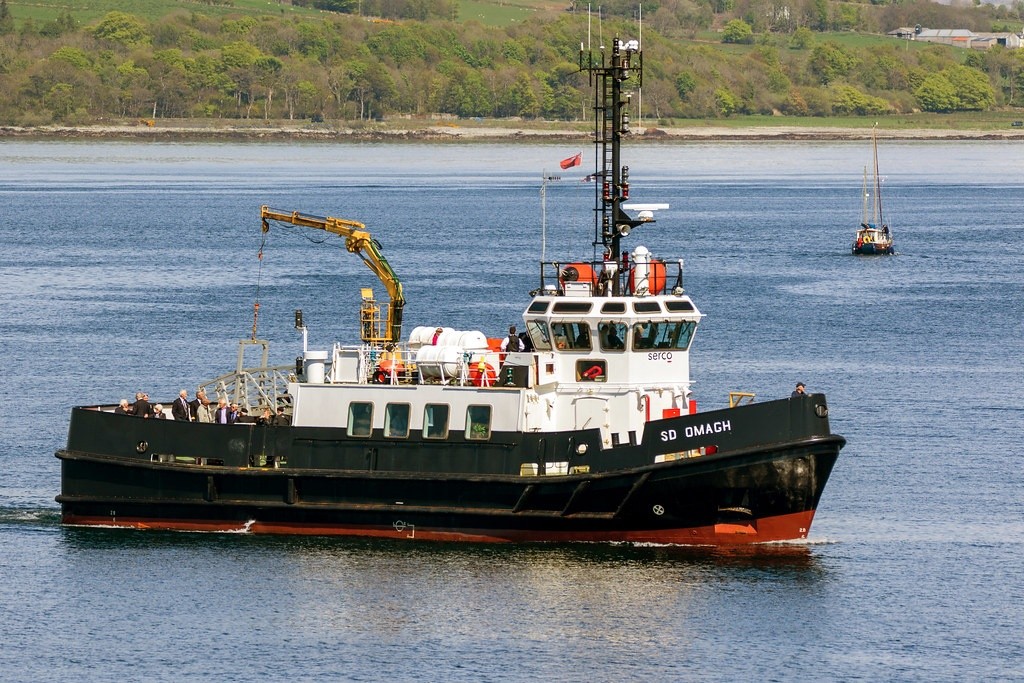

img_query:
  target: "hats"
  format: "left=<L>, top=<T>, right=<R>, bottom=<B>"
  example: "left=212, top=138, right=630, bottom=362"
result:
left=796, top=382, right=806, bottom=387
left=155, top=404, right=162, bottom=412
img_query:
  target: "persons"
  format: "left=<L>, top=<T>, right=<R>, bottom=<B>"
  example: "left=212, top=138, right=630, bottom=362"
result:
left=190, top=388, right=292, bottom=427
left=500, top=326, right=525, bottom=353
left=392, top=407, right=407, bottom=436
left=172, top=390, right=190, bottom=422
left=792, top=382, right=806, bottom=396
left=115, top=393, right=166, bottom=420
left=576, top=325, right=645, bottom=349
left=882, top=225, right=889, bottom=241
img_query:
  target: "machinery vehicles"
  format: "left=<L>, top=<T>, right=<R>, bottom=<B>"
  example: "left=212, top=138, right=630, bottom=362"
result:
left=251, top=202, right=419, bottom=383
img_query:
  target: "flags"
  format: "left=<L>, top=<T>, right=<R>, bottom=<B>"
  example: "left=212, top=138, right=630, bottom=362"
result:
left=560, top=152, right=582, bottom=169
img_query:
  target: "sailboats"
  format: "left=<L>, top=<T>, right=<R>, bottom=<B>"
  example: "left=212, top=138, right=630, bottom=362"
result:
left=847, top=122, right=895, bottom=256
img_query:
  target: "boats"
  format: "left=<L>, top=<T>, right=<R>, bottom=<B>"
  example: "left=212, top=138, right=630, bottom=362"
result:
left=52, top=0, right=850, bottom=558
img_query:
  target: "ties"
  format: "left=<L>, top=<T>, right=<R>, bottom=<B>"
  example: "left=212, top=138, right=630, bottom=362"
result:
left=232, top=413, right=234, bottom=420
left=183, top=401, right=187, bottom=416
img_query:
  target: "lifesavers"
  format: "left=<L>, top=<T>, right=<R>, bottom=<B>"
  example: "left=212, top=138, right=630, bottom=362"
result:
left=858, top=237, right=862, bottom=248
left=583, top=365, right=602, bottom=379
left=863, top=235, right=870, bottom=244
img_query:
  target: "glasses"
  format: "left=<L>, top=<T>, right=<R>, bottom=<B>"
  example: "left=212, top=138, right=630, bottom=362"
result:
left=143, top=395, right=149, bottom=397
